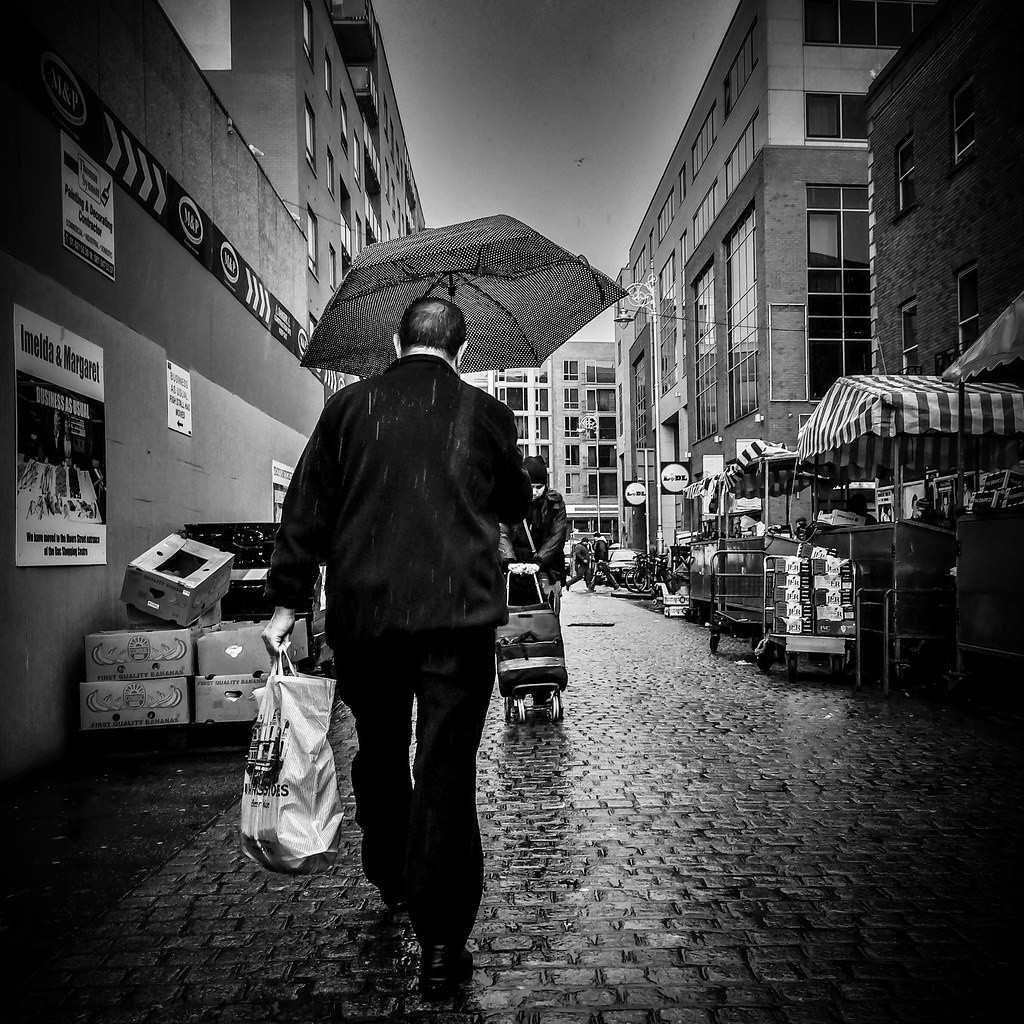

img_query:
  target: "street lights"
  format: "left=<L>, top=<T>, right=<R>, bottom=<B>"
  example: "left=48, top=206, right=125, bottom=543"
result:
left=614, top=258, right=660, bottom=599
left=576, top=404, right=601, bottom=532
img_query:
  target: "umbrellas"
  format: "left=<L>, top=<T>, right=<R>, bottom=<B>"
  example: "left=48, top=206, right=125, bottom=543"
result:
left=296, top=213, right=631, bottom=374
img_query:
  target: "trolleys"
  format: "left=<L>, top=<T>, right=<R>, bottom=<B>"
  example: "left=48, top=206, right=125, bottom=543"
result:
left=708, top=550, right=775, bottom=653
left=756, top=553, right=856, bottom=675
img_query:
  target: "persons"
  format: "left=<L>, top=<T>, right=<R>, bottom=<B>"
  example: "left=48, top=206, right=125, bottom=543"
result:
left=564, top=536, right=597, bottom=592
left=495, top=453, right=568, bottom=717
left=793, top=516, right=807, bottom=531
left=878, top=478, right=972, bottom=524
left=583, top=531, right=622, bottom=594
left=647, top=539, right=657, bottom=555
left=262, top=291, right=533, bottom=996
left=844, top=492, right=877, bottom=525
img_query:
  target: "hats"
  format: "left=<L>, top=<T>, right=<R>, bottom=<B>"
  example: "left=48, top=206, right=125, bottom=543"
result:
left=582, top=537, right=590, bottom=542
left=524, top=456, right=548, bottom=485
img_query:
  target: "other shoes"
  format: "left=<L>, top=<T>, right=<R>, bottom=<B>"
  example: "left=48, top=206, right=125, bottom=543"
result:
left=614, top=586, right=621, bottom=591
left=584, top=588, right=594, bottom=593
left=566, top=582, right=570, bottom=591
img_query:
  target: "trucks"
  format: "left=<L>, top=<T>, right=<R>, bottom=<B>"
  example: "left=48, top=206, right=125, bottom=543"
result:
left=568, top=533, right=613, bottom=560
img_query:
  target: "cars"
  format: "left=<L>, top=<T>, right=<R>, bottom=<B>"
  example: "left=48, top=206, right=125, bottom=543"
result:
left=601, top=548, right=639, bottom=585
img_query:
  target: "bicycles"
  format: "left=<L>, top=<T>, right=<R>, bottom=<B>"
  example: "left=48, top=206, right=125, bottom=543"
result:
left=625, top=552, right=674, bottom=594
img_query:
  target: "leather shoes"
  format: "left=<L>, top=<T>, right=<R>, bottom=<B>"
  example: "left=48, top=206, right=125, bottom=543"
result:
left=421, top=945, right=473, bottom=1000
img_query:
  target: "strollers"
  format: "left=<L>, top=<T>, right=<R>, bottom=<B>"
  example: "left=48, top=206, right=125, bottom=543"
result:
left=500, top=569, right=561, bottom=725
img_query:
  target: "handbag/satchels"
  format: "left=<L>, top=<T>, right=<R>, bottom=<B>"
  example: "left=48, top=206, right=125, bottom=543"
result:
left=595, top=562, right=610, bottom=585
left=240, top=643, right=344, bottom=876
